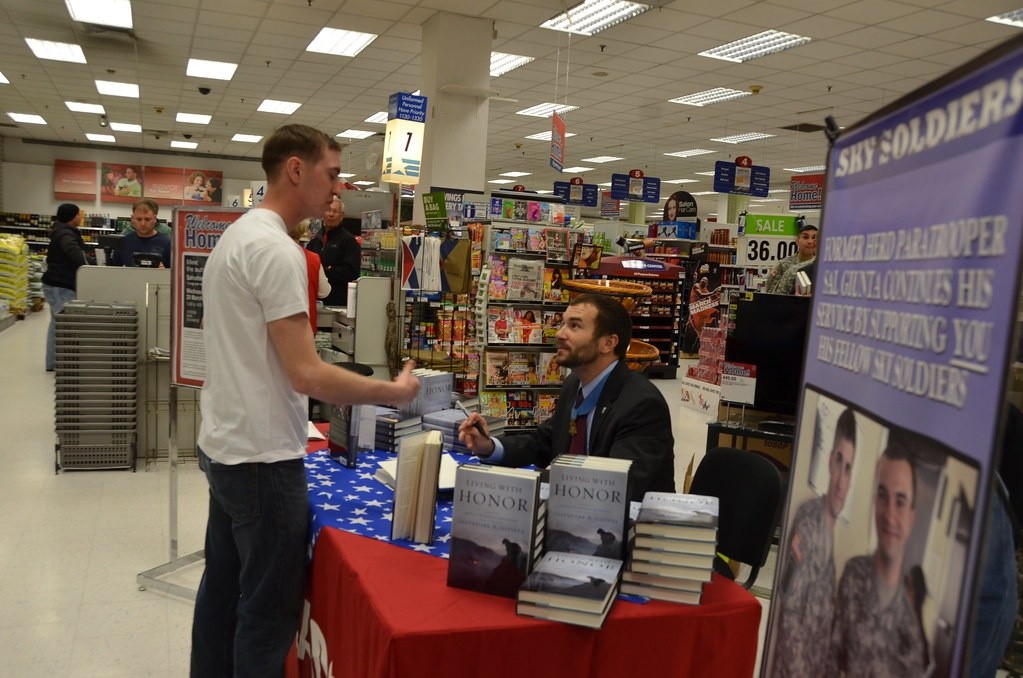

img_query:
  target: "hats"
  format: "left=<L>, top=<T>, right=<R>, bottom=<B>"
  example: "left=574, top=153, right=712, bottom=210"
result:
left=52, top=203, right=79, bottom=223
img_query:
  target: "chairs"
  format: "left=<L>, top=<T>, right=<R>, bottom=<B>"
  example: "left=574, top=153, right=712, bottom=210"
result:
left=688, top=445, right=786, bottom=592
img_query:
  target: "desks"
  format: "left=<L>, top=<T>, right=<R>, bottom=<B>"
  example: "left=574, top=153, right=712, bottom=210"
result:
left=283, top=422, right=762, bottom=678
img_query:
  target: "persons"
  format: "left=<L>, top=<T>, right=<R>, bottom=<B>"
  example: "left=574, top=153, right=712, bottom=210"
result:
left=554, top=312, right=565, bottom=327
left=496, top=312, right=512, bottom=341
left=41, top=203, right=92, bottom=373
left=767, top=224, right=819, bottom=295
left=546, top=356, right=562, bottom=383
left=524, top=362, right=536, bottom=382
left=664, top=197, right=678, bottom=223
left=305, top=197, right=361, bottom=306
left=521, top=311, right=536, bottom=343
left=187, top=123, right=421, bottom=678
left=458, top=293, right=676, bottom=501
left=288, top=217, right=332, bottom=421
left=545, top=315, right=553, bottom=327
left=103, top=166, right=141, bottom=197
left=830, top=441, right=931, bottom=678
left=688, top=277, right=720, bottom=351
left=769, top=408, right=857, bottom=678
left=106, top=200, right=172, bottom=270
left=189, top=171, right=221, bottom=202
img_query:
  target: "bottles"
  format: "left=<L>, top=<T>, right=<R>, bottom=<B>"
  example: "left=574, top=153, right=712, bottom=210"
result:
left=0, top=211, right=110, bottom=262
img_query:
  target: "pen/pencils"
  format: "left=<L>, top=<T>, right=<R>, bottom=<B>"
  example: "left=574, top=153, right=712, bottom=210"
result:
left=456, top=400, right=489, bottom=440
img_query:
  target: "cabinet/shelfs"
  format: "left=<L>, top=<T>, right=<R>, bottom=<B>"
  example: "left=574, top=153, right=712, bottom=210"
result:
left=588, top=256, right=686, bottom=380
left=718, top=264, right=760, bottom=324
left=644, top=238, right=700, bottom=258
left=482, top=223, right=586, bottom=433
left=0, top=224, right=115, bottom=247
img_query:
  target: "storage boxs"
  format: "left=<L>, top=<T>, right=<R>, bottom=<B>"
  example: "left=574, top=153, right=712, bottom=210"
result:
left=477, top=229, right=728, bottom=423
left=51, top=300, right=139, bottom=471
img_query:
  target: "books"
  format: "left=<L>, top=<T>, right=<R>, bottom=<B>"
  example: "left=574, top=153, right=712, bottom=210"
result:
left=447, top=462, right=551, bottom=597
left=390, top=427, right=444, bottom=545
left=795, top=270, right=812, bottom=294
left=479, top=200, right=584, bottom=426
left=328, top=367, right=475, bottom=500
left=706, top=249, right=732, bottom=264
left=704, top=227, right=730, bottom=245
left=620, top=490, right=720, bottom=606
left=718, top=268, right=741, bottom=285
left=545, top=454, right=635, bottom=558
left=516, top=549, right=623, bottom=629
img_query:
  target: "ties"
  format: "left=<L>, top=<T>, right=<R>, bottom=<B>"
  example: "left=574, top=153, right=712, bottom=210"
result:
left=569, top=388, right=589, bottom=455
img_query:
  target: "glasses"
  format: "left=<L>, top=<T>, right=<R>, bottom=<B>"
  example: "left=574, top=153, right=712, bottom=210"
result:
left=327, top=209, right=342, bottom=215
